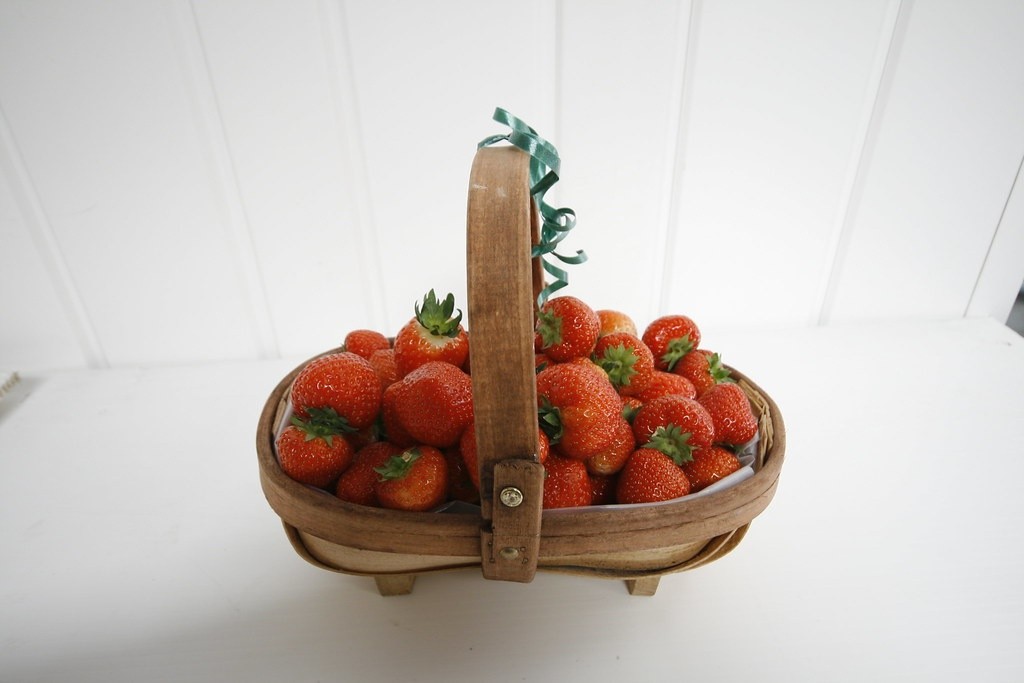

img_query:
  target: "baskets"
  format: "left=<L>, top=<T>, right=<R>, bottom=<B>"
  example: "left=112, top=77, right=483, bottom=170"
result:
left=254, top=144, right=786, bottom=594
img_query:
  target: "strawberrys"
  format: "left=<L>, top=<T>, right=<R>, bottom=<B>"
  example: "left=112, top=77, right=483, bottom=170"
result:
left=272, top=289, right=756, bottom=512
